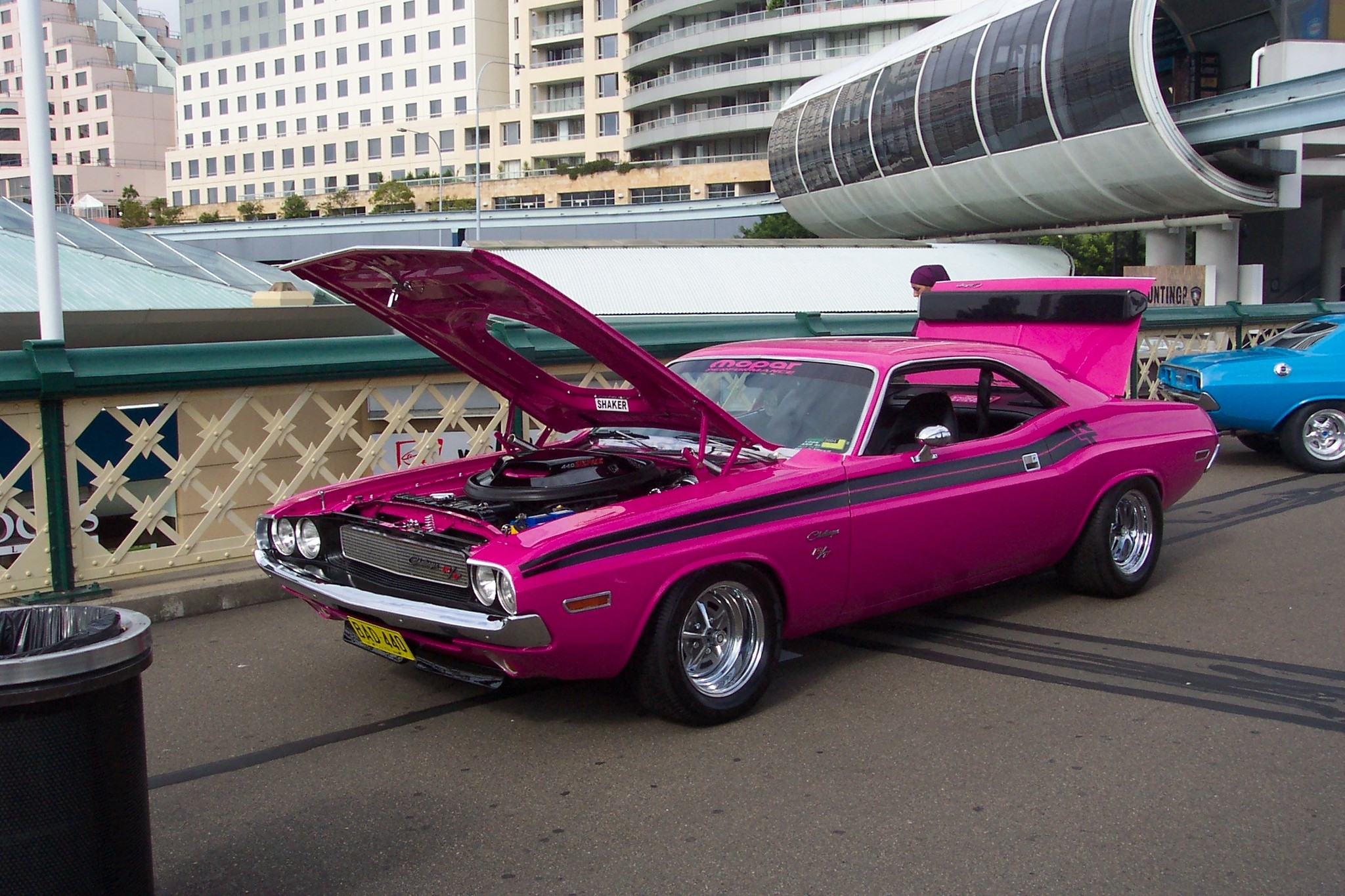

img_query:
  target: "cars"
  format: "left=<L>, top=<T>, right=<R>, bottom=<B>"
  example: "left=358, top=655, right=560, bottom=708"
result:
left=250, top=243, right=1223, bottom=731
left=1156, top=312, right=1345, bottom=475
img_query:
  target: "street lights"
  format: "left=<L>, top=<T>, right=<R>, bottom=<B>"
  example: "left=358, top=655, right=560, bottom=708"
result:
left=19, top=184, right=114, bottom=215
left=475, top=60, right=526, bottom=245
left=397, top=127, right=442, bottom=213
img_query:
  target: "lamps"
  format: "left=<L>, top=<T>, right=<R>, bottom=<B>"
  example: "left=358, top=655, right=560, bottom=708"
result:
left=483, top=202, right=488, bottom=206
left=694, top=189, right=700, bottom=194
left=548, top=198, right=553, bottom=202
left=618, top=193, right=623, bottom=198
left=416, top=206, right=422, bottom=210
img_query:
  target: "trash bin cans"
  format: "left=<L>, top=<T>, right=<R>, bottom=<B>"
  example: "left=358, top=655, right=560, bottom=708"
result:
left=0, top=604, right=155, bottom=896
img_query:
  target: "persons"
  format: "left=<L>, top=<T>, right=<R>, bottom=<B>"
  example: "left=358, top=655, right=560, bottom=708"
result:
left=910, top=265, right=950, bottom=299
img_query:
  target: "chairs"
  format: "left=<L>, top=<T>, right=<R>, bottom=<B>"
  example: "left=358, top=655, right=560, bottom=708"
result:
left=877, top=392, right=959, bottom=455
